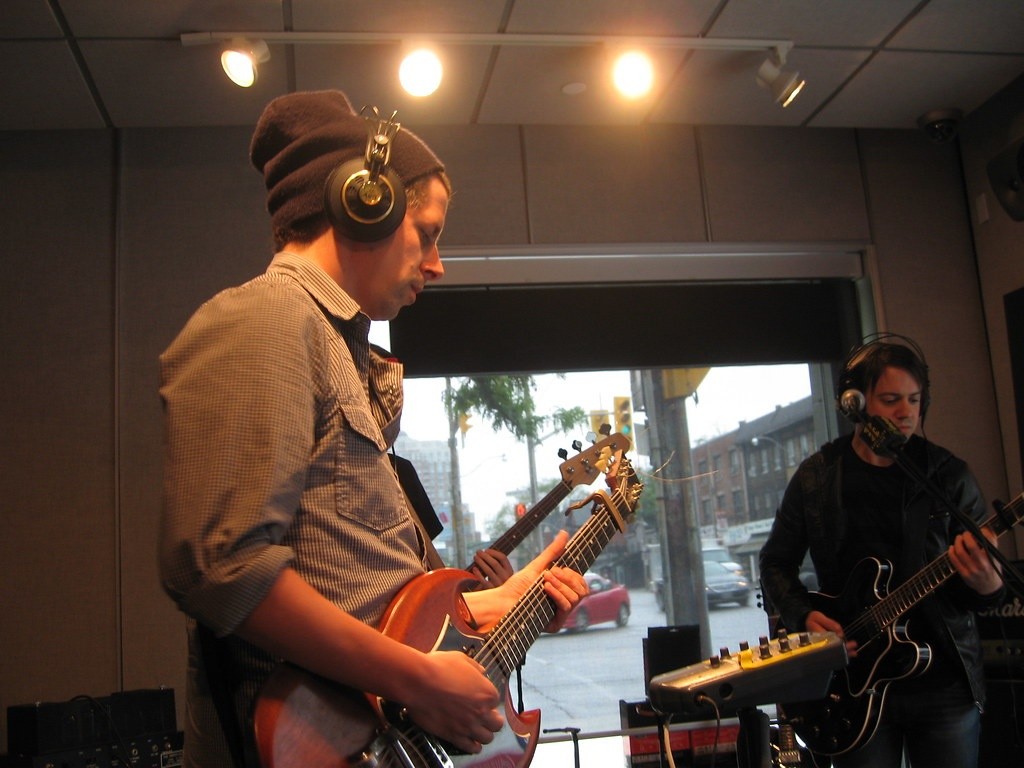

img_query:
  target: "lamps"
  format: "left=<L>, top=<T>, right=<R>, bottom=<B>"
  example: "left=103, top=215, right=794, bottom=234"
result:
left=219, top=32, right=274, bottom=88
left=751, top=49, right=803, bottom=106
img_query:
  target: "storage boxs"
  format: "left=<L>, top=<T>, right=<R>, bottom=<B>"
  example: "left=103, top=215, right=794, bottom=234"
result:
left=618, top=699, right=740, bottom=768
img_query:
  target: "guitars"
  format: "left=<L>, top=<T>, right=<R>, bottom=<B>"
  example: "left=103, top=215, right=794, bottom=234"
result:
left=461, top=422, right=630, bottom=577
left=253, top=445, right=644, bottom=768
left=768, top=493, right=1024, bottom=756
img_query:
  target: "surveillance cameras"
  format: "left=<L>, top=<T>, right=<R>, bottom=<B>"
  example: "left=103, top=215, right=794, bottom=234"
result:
left=916, top=107, right=964, bottom=143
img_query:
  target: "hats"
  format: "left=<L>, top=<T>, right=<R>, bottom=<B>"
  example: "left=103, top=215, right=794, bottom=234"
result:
left=248, top=90, right=446, bottom=235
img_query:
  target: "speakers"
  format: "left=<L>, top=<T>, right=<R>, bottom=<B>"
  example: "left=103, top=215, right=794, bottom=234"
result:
left=978, top=679, right=1024, bottom=768
left=642, top=625, right=702, bottom=695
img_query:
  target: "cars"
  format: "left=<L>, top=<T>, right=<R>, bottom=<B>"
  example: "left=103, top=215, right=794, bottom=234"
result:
left=563, top=572, right=631, bottom=633
left=654, top=559, right=750, bottom=613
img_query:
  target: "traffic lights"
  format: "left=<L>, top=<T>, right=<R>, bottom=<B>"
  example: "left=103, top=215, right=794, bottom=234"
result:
left=515, top=503, right=526, bottom=520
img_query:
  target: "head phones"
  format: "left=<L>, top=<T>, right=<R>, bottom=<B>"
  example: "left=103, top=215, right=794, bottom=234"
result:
left=836, top=331, right=930, bottom=423
left=325, top=103, right=406, bottom=242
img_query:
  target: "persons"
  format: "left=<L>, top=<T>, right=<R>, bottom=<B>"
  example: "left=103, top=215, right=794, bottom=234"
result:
left=154, top=91, right=589, bottom=768
left=760, top=342, right=1004, bottom=768
